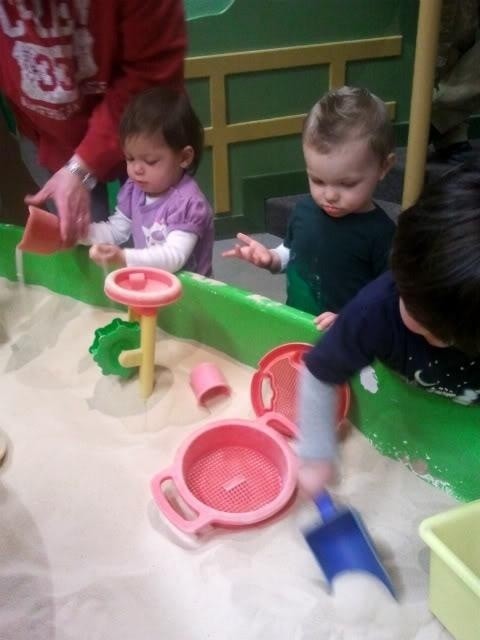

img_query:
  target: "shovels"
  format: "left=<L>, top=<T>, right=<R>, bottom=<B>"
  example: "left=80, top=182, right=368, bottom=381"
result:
left=18, top=205, right=63, bottom=254
left=302, top=490, right=398, bottom=602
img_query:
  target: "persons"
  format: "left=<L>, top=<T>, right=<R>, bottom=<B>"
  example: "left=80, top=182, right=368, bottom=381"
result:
left=76, top=88, right=216, bottom=276
left=294, top=157, right=480, bottom=499
left=220, top=85, right=397, bottom=333
left=0, top=0, right=188, bottom=250
